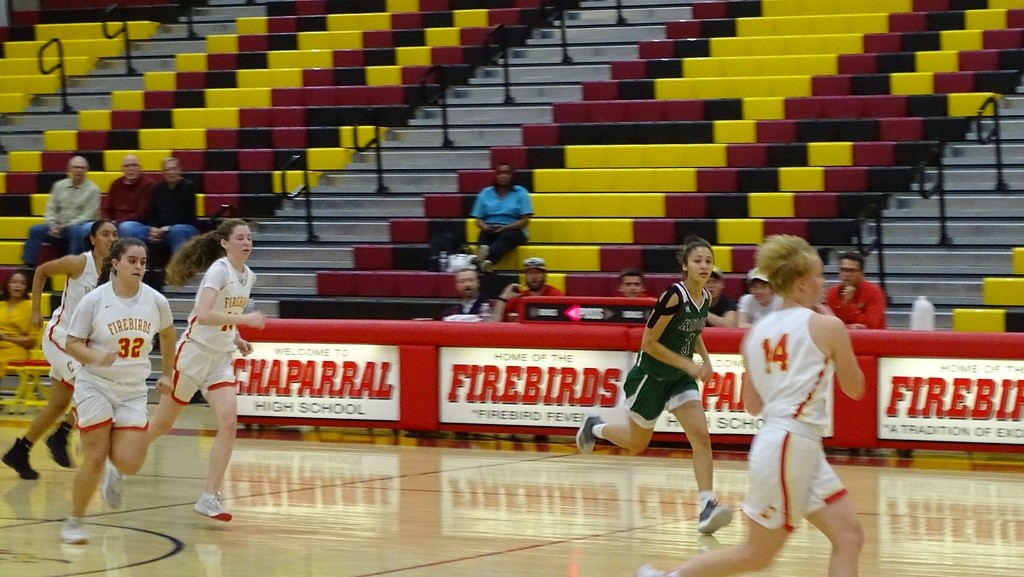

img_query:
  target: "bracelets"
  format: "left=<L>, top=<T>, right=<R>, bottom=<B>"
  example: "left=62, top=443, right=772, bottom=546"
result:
left=496, top=297, right=508, bottom=303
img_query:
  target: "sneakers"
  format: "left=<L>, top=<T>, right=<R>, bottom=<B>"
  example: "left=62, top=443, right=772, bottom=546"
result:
left=45, top=428, right=70, bottom=469
left=697, top=499, right=733, bottom=532
left=194, top=492, right=232, bottom=522
left=3, top=437, right=39, bottom=481
left=102, top=458, right=124, bottom=509
left=60, top=518, right=88, bottom=545
left=576, top=412, right=604, bottom=454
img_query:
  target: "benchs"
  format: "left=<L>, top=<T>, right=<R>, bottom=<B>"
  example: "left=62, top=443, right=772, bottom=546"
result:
left=0, top=0, right=1024, bottom=321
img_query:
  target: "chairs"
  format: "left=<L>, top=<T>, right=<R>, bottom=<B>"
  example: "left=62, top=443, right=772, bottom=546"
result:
left=1, top=322, right=54, bottom=414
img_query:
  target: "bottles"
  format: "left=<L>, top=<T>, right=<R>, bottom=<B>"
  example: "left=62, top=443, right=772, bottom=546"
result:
left=910, top=296, right=936, bottom=330
left=439, top=252, right=447, bottom=272
left=479, top=302, right=492, bottom=322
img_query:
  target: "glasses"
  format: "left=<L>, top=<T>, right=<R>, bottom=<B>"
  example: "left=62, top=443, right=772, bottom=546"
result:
left=839, top=266, right=862, bottom=274
left=525, top=260, right=543, bottom=266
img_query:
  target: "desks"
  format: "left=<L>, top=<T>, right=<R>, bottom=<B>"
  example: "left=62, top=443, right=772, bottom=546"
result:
left=236, top=321, right=1024, bottom=459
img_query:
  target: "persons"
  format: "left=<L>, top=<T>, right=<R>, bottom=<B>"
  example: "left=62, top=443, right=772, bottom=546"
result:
left=826, top=252, right=887, bottom=329
left=0, top=270, right=39, bottom=381
left=147, top=218, right=267, bottom=521
left=440, top=269, right=490, bottom=321
left=471, top=163, right=534, bottom=273
left=636, top=236, right=865, bottom=577
left=2, top=220, right=120, bottom=481
left=490, top=257, right=562, bottom=323
left=21, top=156, right=101, bottom=293
left=120, top=159, right=201, bottom=270
left=78, top=155, right=159, bottom=255
left=60, top=239, right=177, bottom=544
left=737, top=267, right=784, bottom=328
left=704, top=268, right=737, bottom=328
left=575, top=237, right=733, bottom=534
left=617, top=269, right=648, bottom=298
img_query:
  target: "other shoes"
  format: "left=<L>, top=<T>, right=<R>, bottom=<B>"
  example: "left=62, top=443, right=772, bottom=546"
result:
left=470, top=257, right=493, bottom=272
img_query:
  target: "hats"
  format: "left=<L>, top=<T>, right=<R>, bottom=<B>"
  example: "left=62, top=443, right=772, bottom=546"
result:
left=709, top=264, right=721, bottom=279
left=524, top=256, right=548, bottom=272
left=746, top=267, right=770, bottom=283
